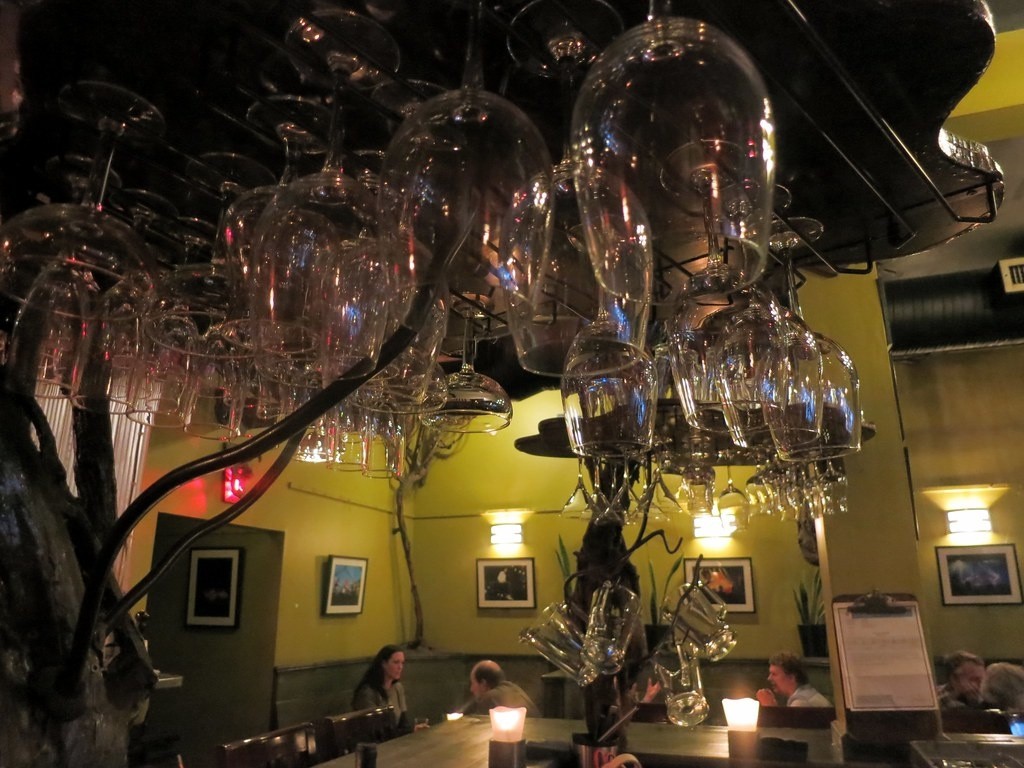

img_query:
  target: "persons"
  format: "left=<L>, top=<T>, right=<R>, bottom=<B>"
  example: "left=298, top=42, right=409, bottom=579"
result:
left=622, top=657, right=662, bottom=703
left=935, top=650, right=1024, bottom=734
left=486, top=567, right=517, bottom=600
left=756, top=650, right=834, bottom=707
left=351, top=645, right=430, bottom=731
left=455, top=660, right=543, bottom=719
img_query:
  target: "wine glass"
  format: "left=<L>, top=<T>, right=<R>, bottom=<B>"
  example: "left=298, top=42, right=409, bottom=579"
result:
left=0, top=0, right=861, bottom=525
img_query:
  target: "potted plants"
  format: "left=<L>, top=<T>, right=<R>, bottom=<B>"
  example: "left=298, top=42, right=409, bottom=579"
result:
left=792, top=567, right=827, bottom=659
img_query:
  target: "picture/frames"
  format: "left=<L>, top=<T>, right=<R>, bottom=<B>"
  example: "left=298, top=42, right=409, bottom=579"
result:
left=322, top=555, right=369, bottom=615
left=683, top=557, right=756, bottom=614
left=935, top=544, right=1024, bottom=607
left=184, top=547, right=244, bottom=629
left=476, top=557, right=537, bottom=609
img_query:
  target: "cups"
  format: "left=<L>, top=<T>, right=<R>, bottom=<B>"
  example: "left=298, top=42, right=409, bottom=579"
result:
left=517, top=584, right=641, bottom=687
left=651, top=583, right=739, bottom=727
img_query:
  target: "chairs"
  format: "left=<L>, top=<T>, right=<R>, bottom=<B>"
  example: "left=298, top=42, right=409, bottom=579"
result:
left=148, top=706, right=398, bottom=768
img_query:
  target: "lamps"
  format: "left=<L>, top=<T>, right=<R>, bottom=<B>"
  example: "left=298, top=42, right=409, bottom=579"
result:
left=489, top=524, right=523, bottom=544
left=489, top=705, right=529, bottom=768
left=946, top=506, right=994, bottom=534
left=693, top=514, right=732, bottom=538
left=721, top=699, right=759, bottom=757
